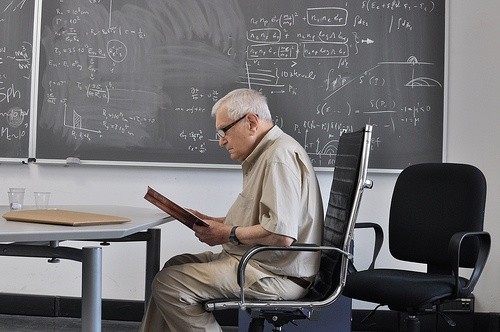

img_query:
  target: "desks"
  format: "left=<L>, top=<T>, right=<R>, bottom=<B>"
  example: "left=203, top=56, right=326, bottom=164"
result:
left=0, top=205, right=177, bottom=332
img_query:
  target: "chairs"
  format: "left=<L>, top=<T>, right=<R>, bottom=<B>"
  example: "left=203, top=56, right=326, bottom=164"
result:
left=201, top=124, right=374, bottom=332
left=341, top=162, right=491, bottom=332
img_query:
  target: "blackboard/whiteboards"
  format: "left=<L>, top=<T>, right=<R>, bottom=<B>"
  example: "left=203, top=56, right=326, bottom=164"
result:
left=0, top=0, right=39, bottom=162
left=30, top=0, right=448, bottom=173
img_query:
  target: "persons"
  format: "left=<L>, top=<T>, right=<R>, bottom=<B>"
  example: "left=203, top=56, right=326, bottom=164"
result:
left=138, top=88, right=324, bottom=332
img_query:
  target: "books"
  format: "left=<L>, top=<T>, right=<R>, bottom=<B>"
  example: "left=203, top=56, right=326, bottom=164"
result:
left=144, top=185, right=210, bottom=232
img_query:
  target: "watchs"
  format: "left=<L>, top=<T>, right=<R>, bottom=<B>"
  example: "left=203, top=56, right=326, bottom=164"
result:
left=229, top=226, right=241, bottom=246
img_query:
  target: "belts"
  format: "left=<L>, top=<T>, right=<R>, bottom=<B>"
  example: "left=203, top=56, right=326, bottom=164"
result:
left=282, top=275, right=314, bottom=289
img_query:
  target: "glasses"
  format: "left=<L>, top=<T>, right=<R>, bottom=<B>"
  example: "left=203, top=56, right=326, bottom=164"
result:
left=215, top=113, right=258, bottom=140
